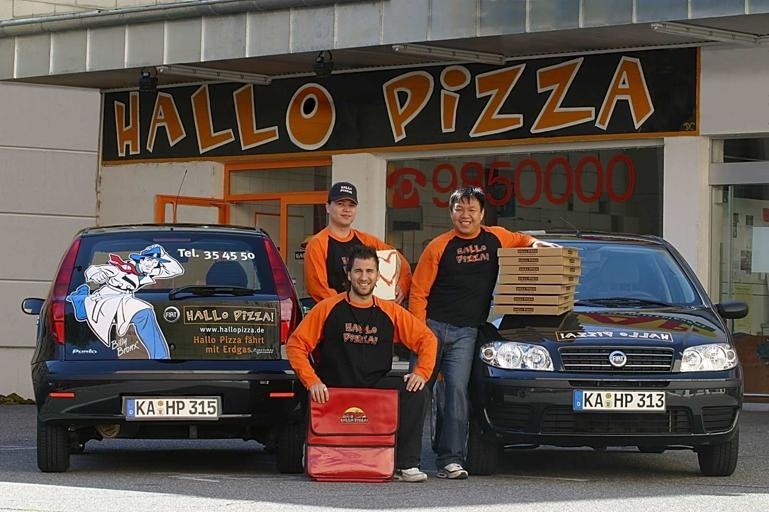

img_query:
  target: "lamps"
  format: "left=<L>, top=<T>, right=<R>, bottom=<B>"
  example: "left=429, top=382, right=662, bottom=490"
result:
left=651, top=21, right=758, bottom=48
left=313, top=50, right=335, bottom=78
left=139, top=66, right=158, bottom=92
left=156, top=65, right=272, bottom=86
left=392, top=44, right=505, bottom=66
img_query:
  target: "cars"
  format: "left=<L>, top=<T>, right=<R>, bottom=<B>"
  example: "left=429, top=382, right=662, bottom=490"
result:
left=429, top=230, right=748, bottom=476
left=20, top=221, right=316, bottom=475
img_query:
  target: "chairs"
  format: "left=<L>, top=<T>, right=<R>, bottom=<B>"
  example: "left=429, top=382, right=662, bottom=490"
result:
left=599, top=253, right=643, bottom=297
left=206, top=261, right=248, bottom=287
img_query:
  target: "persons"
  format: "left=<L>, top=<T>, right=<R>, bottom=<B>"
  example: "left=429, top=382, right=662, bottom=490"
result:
left=287, top=245, right=441, bottom=484
left=408, top=183, right=564, bottom=479
left=303, top=182, right=413, bottom=311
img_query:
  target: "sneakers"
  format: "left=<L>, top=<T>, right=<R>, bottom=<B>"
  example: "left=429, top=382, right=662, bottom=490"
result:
left=437, top=463, right=468, bottom=479
left=395, top=466, right=426, bottom=483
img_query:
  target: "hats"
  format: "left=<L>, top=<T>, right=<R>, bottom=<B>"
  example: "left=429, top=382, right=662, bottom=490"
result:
left=329, top=182, right=358, bottom=205
left=131, top=246, right=169, bottom=265
